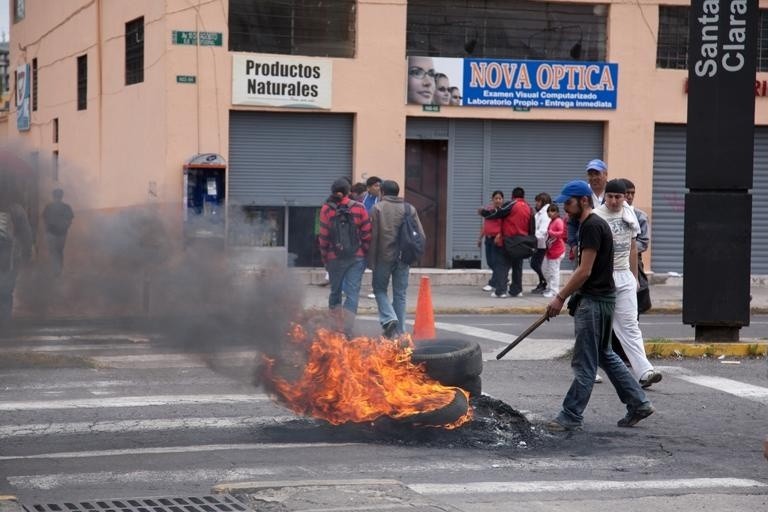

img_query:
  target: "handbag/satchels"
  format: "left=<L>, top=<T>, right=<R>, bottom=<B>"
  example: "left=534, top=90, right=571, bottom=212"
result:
left=503, top=235, right=538, bottom=260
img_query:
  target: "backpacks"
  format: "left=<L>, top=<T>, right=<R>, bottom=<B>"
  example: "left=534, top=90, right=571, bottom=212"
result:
left=395, top=202, right=427, bottom=264
left=326, top=200, right=361, bottom=259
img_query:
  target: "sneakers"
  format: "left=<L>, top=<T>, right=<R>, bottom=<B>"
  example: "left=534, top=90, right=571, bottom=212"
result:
left=638, top=370, right=663, bottom=389
left=483, top=285, right=522, bottom=298
left=616, top=403, right=655, bottom=427
left=547, top=420, right=568, bottom=431
left=383, top=320, right=396, bottom=339
left=532, top=287, right=557, bottom=297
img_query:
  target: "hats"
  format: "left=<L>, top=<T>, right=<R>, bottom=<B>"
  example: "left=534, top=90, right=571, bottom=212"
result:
left=553, top=179, right=591, bottom=204
left=585, top=158, right=607, bottom=173
left=605, top=179, right=627, bottom=194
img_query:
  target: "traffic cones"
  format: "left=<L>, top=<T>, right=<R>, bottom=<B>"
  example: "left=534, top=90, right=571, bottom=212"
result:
left=412, top=276, right=436, bottom=340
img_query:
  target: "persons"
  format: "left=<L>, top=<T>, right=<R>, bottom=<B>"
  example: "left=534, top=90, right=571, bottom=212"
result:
left=368, top=180, right=427, bottom=339
left=449, top=87, right=461, bottom=106
left=351, top=177, right=383, bottom=273
left=319, top=177, right=372, bottom=342
left=478, top=186, right=536, bottom=297
left=434, top=73, right=451, bottom=104
left=530, top=192, right=553, bottom=293
left=566, top=217, right=581, bottom=272
left=43, top=188, right=73, bottom=270
left=476, top=190, right=504, bottom=291
left=540, top=203, right=567, bottom=297
left=592, top=179, right=663, bottom=388
left=546, top=180, right=656, bottom=431
left=1, top=189, right=34, bottom=326
left=408, top=57, right=435, bottom=105
left=587, top=159, right=611, bottom=209
